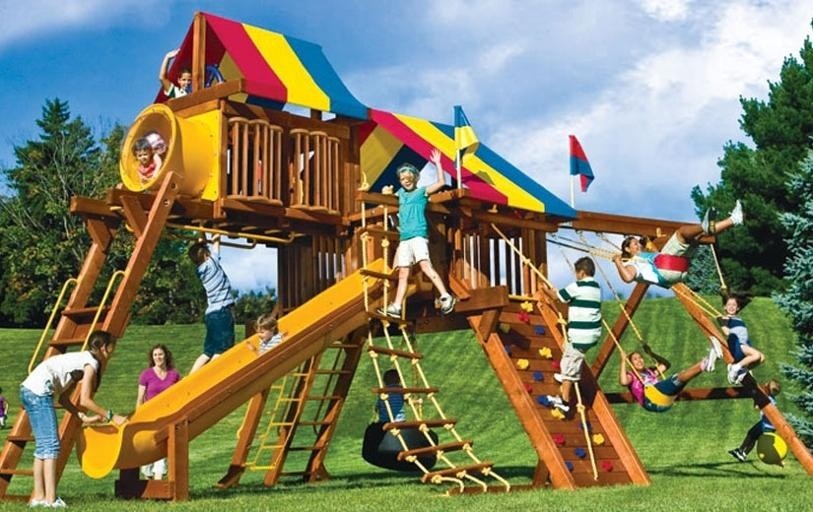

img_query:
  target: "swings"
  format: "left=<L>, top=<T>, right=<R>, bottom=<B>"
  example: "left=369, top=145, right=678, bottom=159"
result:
left=598, top=233, right=751, bottom=362
left=541, top=233, right=690, bottom=286
left=709, top=244, right=789, bottom=463
left=553, top=233, right=679, bottom=413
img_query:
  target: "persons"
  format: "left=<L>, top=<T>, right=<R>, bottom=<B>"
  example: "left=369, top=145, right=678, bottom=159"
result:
left=541, top=257, right=601, bottom=412
left=369, top=369, right=422, bottom=427
left=728, top=382, right=781, bottom=463
left=717, top=293, right=765, bottom=385
left=184, top=221, right=235, bottom=379
left=612, top=199, right=745, bottom=291
left=19, top=329, right=128, bottom=507
left=131, top=138, right=156, bottom=183
left=158, top=48, right=192, bottom=100
left=143, top=130, right=169, bottom=176
left=136, top=342, right=181, bottom=480
left=619, top=335, right=723, bottom=412
left=247, top=315, right=286, bottom=360
left=0, top=388, right=9, bottom=429
left=376, top=148, right=456, bottom=319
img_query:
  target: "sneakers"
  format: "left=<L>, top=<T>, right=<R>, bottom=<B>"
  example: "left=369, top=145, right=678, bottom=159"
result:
left=375, top=302, right=402, bottom=319
left=554, top=373, right=564, bottom=384
left=547, top=394, right=571, bottom=412
left=440, top=293, right=457, bottom=315
left=708, top=335, right=724, bottom=361
left=726, top=362, right=748, bottom=386
left=728, top=447, right=748, bottom=462
left=703, top=348, right=717, bottom=373
left=702, top=205, right=718, bottom=236
left=30, top=496, right=70, bottom=509
left=733, top=198, right=746, bottom=226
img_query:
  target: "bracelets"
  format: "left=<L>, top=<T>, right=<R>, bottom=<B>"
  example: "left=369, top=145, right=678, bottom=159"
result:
left=107, top=409, right=112, bottom=422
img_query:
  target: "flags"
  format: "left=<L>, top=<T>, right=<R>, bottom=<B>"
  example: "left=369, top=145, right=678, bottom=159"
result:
left=453, top=105, right=478, bottom=168
left=569, top=134, right=595, bottom=193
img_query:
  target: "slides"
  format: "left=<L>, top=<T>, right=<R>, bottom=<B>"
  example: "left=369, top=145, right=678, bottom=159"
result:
left=73, top=258, right=434, bottom=480
